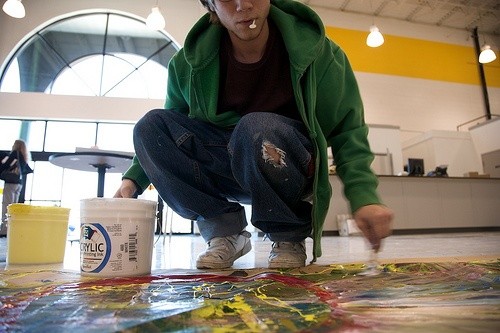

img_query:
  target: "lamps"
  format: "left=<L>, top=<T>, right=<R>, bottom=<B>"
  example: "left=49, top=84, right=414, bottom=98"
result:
left=2, top=0, right=26, bottom=19
left=477, top=8, right=497, bottom=63
left=366, top=1, right=384, bottom=47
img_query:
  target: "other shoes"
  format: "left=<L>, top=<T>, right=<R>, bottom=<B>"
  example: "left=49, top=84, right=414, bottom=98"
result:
left=0, top=234, right=7, bottom=238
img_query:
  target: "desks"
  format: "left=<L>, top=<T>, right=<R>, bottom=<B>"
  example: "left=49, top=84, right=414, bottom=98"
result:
left=323, top=173, right=500, bottom=235
left=48, top=153, right=134, bottom=197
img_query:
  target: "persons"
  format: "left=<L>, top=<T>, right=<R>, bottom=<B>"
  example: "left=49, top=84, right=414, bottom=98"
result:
left=113, top=0, right=394, bottom=270
left=0, top=140, right=26, bottom=237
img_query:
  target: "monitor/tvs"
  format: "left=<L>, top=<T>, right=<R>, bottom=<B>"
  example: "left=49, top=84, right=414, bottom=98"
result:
left=408, top=158, right=424, bottom=175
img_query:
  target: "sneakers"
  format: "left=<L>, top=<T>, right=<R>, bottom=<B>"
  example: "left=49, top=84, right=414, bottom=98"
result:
left=268, top=240, right=307, bottom=267
left=196, top=226, right=252, bottom=269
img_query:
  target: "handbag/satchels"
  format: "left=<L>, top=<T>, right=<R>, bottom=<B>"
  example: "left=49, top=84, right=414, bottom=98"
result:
left=0, top=152, right=20, bottom=184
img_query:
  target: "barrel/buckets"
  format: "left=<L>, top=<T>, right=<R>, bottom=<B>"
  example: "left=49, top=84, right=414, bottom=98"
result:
left=6, top=203, right=71, bottom=265
left=80, top=198, right=162, bottom=277
